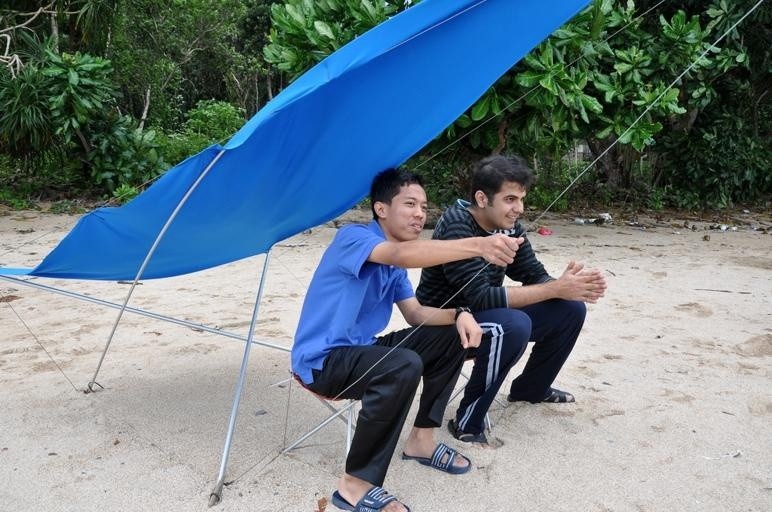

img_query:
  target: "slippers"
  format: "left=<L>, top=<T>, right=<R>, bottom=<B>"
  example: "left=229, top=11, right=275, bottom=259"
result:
left=447, top=419, right=489, bottom=447
left=332, top=485, right=410, bottom=512
left=507, top=388, right=575, bottom=403
left=401, top=443, right=471, bottom=474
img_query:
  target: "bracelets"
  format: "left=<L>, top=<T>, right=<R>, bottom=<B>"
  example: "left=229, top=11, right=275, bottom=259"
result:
left=452, top=306, right=474, bottom=322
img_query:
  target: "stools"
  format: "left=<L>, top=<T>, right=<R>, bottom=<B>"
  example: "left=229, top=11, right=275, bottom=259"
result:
left=281, top=368, right=362, bottom=470
left=445, top=356, right=507, bottom=434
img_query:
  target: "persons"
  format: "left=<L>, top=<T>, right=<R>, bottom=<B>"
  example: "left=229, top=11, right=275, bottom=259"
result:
left=290, top=164, right=526, bottom=512
left=412, top=152, right=608, bottom=450
